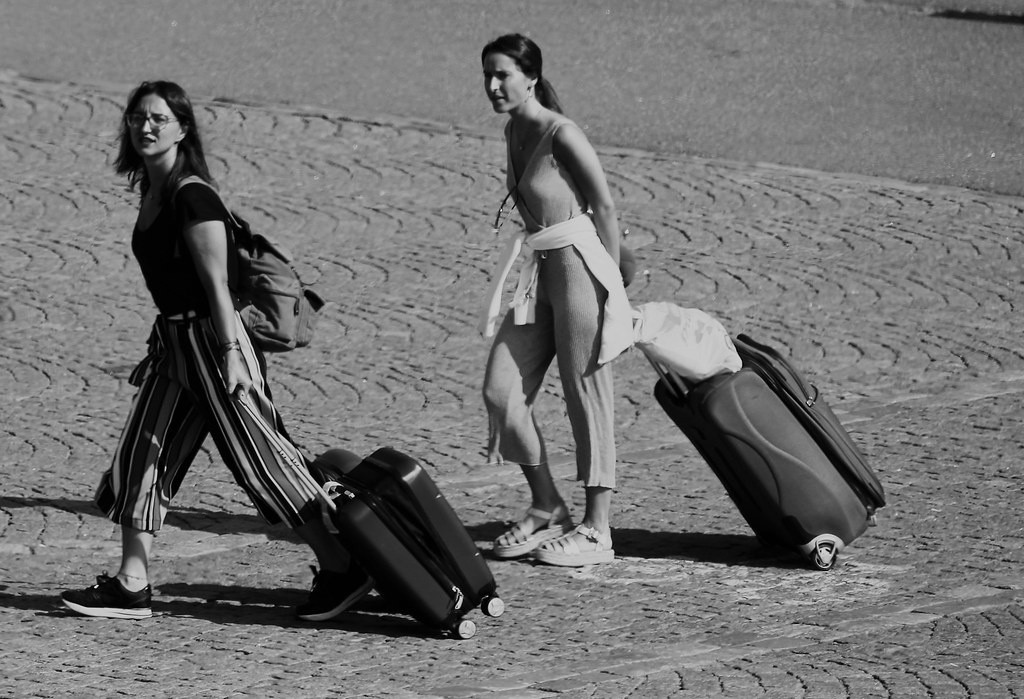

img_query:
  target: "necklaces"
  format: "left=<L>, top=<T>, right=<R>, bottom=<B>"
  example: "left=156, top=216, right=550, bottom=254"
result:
left=509, top=104, right=544, bottom=152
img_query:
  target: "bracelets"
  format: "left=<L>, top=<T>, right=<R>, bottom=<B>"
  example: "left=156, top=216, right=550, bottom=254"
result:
left=220, top=341, right=243, bottom=351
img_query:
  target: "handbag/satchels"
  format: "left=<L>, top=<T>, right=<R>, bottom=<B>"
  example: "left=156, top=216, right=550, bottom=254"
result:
left=632, top=302, right=744, bottom=381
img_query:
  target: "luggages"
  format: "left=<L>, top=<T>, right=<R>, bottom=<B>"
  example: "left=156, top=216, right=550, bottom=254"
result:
left=631, top=298, right=885, bottom=571
left=232, top=383, right=504, bottom=640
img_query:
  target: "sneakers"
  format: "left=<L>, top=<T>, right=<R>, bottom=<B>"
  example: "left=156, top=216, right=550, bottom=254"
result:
left=297, top=566, right=374, bottom=622
left=60, top=570, right=153, bottom=620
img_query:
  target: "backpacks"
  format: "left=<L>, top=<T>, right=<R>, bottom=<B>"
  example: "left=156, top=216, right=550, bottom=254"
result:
left=172, top=174, right=329, bottom=352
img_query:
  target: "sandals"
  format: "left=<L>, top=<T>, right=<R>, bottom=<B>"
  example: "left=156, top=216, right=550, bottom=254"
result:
left=493, top=501, right=574, bottom=558
left=534, top=523, right=614, bottom=567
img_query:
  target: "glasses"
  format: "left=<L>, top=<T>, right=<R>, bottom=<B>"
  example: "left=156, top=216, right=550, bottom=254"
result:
left=493, top=183, right=520, bottom=232
left=124, top=112, right=178, bottom=129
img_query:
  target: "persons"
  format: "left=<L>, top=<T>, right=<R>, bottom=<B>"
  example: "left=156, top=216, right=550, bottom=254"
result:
left=60, top=79, right=376, bottom=620
left=480, top=32, right=636, bottom=568
